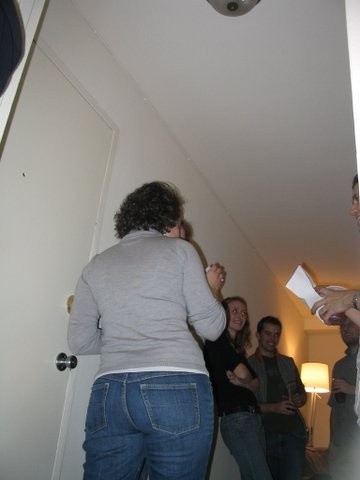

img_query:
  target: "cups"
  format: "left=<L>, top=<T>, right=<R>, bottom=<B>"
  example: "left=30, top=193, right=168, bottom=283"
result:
left=335, top=391, right=347, bottom=403
left=326, top=286, right=349, bottom=324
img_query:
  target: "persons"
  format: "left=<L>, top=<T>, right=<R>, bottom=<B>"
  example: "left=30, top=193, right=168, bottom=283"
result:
left=67, top=182, right=228, bottom=480
left=326, top=319, right=360, bottom=480
left=246, top=316, right=309, bottom=480
left=310, top=172, right=360, bottom=424
left=204, top=296, right=273, bottom=480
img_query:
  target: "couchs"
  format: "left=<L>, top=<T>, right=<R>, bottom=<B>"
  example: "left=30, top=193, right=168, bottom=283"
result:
left=306, top=448, right=330, bottom=479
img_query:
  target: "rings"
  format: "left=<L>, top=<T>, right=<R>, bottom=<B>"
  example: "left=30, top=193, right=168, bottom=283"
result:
left=332, top=379, right=335, bottom=382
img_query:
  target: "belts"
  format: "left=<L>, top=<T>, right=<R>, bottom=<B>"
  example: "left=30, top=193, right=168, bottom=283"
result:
left=219, top=406, right=262, bottom=417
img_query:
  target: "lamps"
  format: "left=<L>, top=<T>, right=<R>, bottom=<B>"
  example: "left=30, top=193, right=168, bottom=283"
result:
left=300, top=363, right=329, bottom=446
